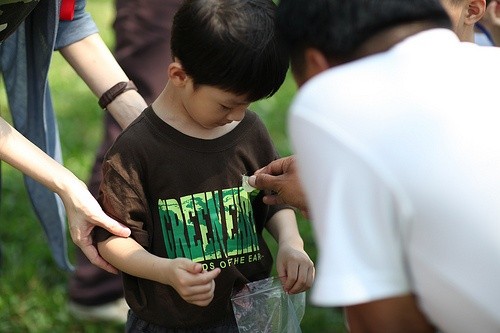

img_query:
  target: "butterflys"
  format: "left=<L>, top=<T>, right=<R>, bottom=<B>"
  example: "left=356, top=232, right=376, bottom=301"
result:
left=238, top=171, right=260, bottom=197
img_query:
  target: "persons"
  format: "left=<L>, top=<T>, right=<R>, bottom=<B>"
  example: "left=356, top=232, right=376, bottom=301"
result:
left=90, top=0, right=315, bottom=333
left=438, top=0, right=486, bottom=44
left=474, top=0, right=500, bottom=48
left=248, top=0, right=500, bottom=333
left=1, top=0, right=149, bottom=278
left=66, top=0, right=187, bottom=323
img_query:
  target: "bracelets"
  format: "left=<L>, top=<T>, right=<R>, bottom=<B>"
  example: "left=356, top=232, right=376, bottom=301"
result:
left=98, top=81, right=137, bottom=109
